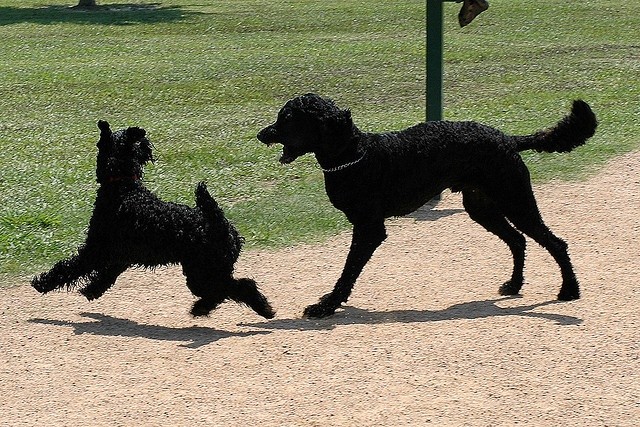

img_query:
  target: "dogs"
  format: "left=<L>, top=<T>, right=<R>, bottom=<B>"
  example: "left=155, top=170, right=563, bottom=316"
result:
left=255, top=90, right=598, bottom=322
left=27, top=91, right=277, bottom=321
